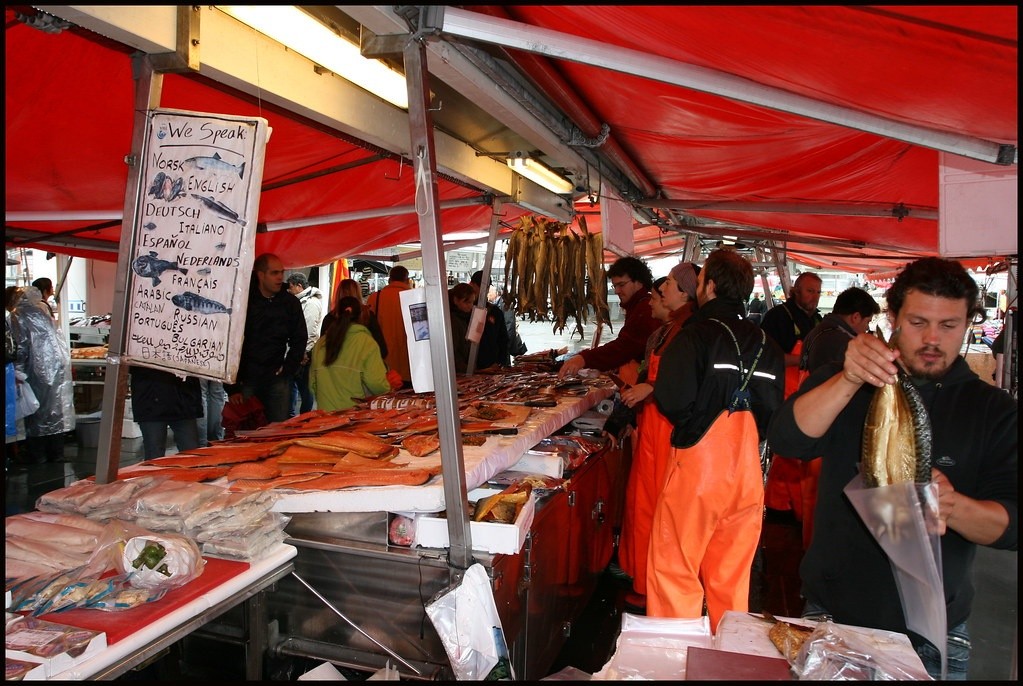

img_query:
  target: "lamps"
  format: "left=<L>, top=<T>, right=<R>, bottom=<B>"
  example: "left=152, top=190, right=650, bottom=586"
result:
left=216, top=4, right=441, bottom=111
left=507, top=155, right=575, bottom=194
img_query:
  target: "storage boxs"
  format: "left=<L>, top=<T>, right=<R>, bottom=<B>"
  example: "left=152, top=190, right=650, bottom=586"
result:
left=416, top=486, right=534, bottom=553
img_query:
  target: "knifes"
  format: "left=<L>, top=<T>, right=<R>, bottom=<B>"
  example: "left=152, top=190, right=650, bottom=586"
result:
left=478, top=400, right=557, bottom=407
left=461, top=428, right=518, bottom=435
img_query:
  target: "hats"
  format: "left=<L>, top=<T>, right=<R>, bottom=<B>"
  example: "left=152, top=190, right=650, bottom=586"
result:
left=472, top=270, right=492, bottom=285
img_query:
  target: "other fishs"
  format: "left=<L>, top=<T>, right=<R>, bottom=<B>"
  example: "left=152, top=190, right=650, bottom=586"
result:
left=749, top=606, right=847, bottom=670
left=863, top=369, right=942, bottom=541
left=83, top=363, right=598, bottom=490
left=498, top=213, right=617, bottom=344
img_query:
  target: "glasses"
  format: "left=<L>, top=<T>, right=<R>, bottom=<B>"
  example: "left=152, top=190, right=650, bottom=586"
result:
left=612, top=279, right=631, bottom=290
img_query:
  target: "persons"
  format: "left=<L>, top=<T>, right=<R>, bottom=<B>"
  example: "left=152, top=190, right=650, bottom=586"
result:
left=646, top=249, right=763, bottom=635
left=602, top=277, right=668, bottom=490
left=557, top=257, right=660, bottom=378
left=129, top=253, right=528, bottom=517
left=310, top=296, right=390, bottom=412
left=748, top=293, right=762, bottom=313
left=747, top=272, right=823, bottom=547
left=788, top=288, right=880, bottom=618
left=6, top=278, right=75, bottom=516
left=618, top=262, right=700, bottom=609
left=766, top=257, right=1018, bottom=681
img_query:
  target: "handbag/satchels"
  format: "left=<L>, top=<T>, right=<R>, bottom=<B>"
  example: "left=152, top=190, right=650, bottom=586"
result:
left=15, top=370, right=40, bottom=421
left=221, top=395, right=265, bottom=439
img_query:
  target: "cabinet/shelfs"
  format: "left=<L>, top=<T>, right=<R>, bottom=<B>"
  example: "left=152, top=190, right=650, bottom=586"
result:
left=203, top=427, right=627, bottom=676
